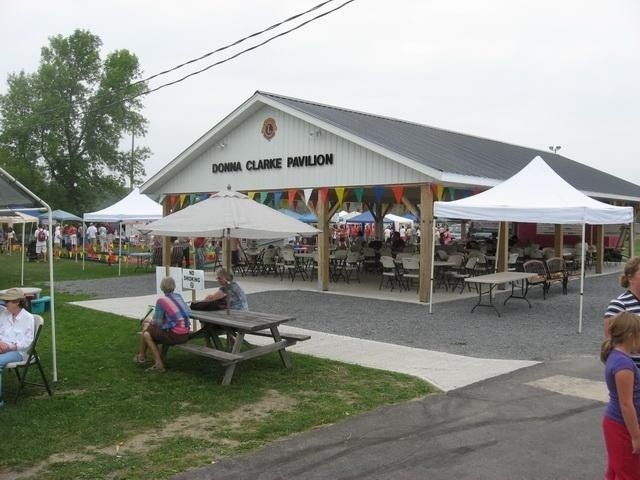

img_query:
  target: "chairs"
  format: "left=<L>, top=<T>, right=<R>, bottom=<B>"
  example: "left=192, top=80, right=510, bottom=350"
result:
left=4, top=315, right=53, bottom=405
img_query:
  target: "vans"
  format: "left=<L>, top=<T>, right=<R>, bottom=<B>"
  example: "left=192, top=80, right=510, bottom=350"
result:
left=451, top=223, right=470, bottom=239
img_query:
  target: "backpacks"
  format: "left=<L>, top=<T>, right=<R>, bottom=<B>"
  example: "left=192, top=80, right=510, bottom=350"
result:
left=37, top=229, right=46, bottom=242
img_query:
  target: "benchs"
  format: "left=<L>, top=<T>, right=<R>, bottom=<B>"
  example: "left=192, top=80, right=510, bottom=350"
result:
left=173, top=344, right=244, bottom=362
left=245, top=330, right=311, bottom=341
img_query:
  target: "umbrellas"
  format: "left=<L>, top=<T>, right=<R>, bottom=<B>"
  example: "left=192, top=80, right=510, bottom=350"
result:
left=138, top=185, right=319, bottom=273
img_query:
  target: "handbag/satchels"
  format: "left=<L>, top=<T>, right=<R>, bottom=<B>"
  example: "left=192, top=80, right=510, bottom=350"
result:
left=190, top=301, right=228, bottom=310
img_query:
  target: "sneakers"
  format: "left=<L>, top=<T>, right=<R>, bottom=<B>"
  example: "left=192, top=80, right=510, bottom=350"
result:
left=144, top=364, right=166, bottom=374
left=132, top=355, right=146, bottom=367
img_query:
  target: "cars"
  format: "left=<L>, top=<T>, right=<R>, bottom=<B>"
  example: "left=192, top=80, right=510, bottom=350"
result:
left=471, top=232, right=493, bottom=243
left=96, top=231, right=126, bottom=245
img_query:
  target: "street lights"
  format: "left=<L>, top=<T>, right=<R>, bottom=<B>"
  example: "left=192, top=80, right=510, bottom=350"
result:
left=125, top=118, right=135, bottom=192
left=549, top=145, right=562, bottom=154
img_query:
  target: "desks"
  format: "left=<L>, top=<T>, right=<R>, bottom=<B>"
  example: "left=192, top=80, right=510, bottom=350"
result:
left=0, top=287, right=42, bottom=308
left=148, top=302, right=311, bottom=386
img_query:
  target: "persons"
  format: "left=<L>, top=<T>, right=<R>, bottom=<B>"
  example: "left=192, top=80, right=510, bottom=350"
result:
left=599, top=311, right=639, bottom=479
left=191, top=266, right=249, bottom=336
left=132, top=276, right=191, bottom=374
left=0, top=287, right=37, bottom=409
left=602, top=255, right=640, bottom=373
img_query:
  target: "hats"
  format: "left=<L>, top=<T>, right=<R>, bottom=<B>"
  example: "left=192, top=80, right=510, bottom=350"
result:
left=0, top=288, right=26, bottom=302
